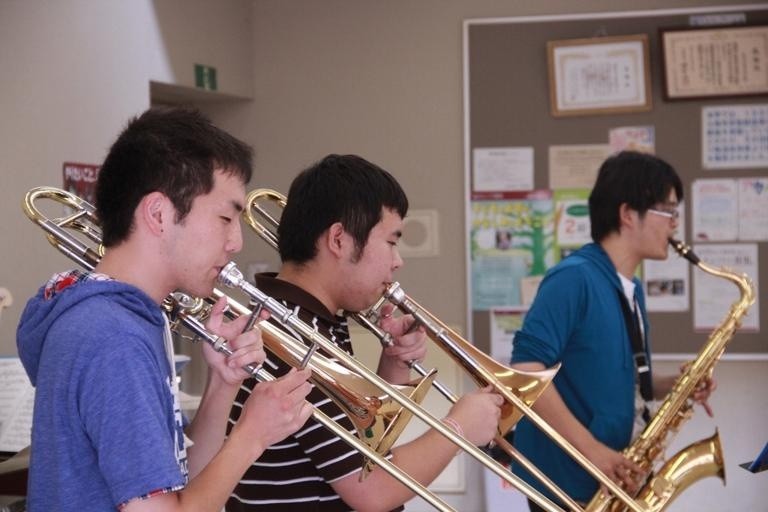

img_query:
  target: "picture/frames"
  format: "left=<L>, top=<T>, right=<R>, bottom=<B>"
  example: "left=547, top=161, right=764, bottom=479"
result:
left=544, top=20, right=768, bottom=118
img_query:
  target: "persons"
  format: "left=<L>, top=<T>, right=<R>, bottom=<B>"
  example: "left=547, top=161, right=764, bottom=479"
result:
left=15, top=105, right=316, bottom=511
left=508, top=150, right=719, bottom=511
left=225, top=153, right=506, bottom=512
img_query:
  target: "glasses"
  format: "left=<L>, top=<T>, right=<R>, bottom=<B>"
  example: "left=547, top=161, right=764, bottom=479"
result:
left=647, top=208, right=678, bottom=221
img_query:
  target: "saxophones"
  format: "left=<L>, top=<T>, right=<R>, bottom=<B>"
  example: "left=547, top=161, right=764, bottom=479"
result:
left=583, top=236, right=755, bottom=511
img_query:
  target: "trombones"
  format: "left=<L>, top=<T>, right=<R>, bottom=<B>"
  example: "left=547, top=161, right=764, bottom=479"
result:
left=23, top=186, right=564, bottom=511
left=242, top=187, right=645, bottom=512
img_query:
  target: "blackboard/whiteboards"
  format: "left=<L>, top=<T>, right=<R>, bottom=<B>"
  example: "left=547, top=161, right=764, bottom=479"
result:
left=461, top=4, right=768, bottom=366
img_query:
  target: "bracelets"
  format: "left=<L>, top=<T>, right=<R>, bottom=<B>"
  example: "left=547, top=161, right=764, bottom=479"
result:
left=440, top=416, right=466, bottom=456
